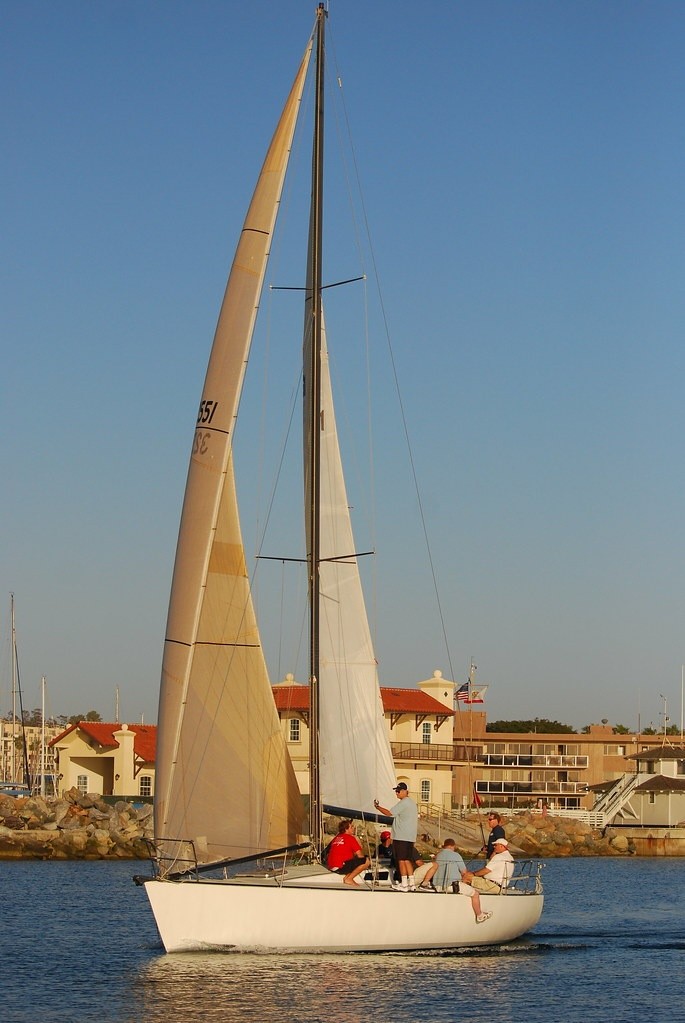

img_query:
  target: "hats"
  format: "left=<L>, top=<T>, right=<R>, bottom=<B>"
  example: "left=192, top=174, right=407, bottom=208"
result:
left=393, top=782, right=407, bottom=791
left=492, top=838, right=509, bottom=848
left=380, top=831, right=390, bottom=839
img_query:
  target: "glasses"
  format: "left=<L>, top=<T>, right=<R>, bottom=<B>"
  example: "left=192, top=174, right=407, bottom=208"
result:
left=493, top=843, right=498, bottom=846
left=395, top=789, right=401, bottom=793
left=489, top=818, right=494, bottom=822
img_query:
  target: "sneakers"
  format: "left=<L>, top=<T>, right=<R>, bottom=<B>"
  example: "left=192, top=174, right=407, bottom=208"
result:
left=410, top=885, right=416, bottom=892
left=392, top=882, right=408, bottom=892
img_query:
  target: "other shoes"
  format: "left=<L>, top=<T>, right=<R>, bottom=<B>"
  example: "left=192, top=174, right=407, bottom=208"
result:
left=419, top=884, right=435, bottom=892
left=477, top=911, right=493, bottom=923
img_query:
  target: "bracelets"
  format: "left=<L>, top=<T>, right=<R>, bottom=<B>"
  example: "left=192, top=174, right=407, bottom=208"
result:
left=473, top=872, right=476, bottom=876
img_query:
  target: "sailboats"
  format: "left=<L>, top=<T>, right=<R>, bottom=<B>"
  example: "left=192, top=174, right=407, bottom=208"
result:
left=0, top=586, right=80, bottom=803
left=130, top=2, right=545, bottom=956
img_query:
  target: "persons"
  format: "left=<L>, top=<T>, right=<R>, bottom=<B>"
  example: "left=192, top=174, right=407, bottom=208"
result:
left=323, top=818, right=370, bottom=887
left=374, top=831, right=396, bottom=868
left=374, top=783, right=417, bottom=892
left=463, top=838, right=514, bottom=892
left=432, top=839, right=493, bottom=923
left=483, top=813, right=506, bottom=865
left=407, top=847, right=438, bottom=892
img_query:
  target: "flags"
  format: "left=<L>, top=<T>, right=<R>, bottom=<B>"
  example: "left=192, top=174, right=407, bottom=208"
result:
left=454, top=683, right=468, bottom=700
left=464, top=687, right=487, bottom=703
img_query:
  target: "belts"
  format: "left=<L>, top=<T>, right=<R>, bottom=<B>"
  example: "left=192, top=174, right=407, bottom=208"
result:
left=484, top=878, right=506, bottom=889
